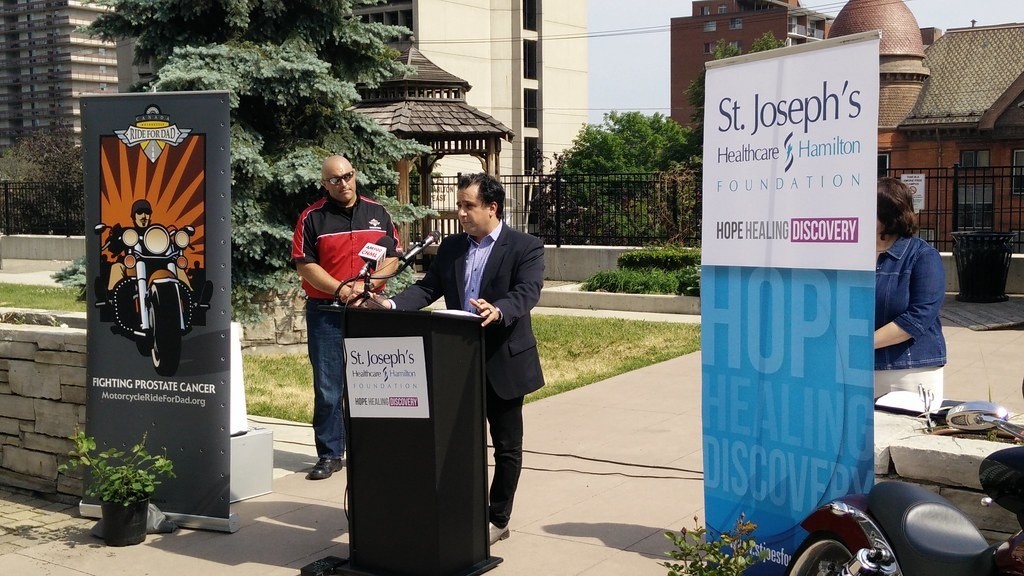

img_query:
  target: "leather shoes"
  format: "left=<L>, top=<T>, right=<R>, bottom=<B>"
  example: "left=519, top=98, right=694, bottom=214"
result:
left=309, top=457, right=342, bottom=479
left=488, top=520, right=510, bottom=547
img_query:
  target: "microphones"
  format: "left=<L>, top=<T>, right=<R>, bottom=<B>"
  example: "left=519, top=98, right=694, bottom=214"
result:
left=403, top=230, right=441, bottom=261
left=358, top=236, right=394, bottom=279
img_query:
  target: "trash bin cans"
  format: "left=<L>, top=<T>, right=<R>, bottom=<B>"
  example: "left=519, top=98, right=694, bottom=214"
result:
left=949, top=230, right=1019, bottom=304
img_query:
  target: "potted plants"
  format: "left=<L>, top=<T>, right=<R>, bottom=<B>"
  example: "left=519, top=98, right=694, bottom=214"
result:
left=57, top=423, right=176, bottom=546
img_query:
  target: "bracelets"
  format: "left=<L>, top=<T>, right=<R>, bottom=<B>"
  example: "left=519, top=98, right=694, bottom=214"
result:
left=493, top=304, right=501, bottom=322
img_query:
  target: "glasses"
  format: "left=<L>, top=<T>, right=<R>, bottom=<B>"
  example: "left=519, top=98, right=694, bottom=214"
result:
left=322, top=170, right=354, bottom=186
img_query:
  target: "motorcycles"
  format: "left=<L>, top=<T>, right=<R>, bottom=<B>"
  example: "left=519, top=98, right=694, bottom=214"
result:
left=785, top=400, right=1023, bottom=576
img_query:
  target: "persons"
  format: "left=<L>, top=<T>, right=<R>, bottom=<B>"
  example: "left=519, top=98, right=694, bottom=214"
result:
left=291, top=155, right=405, bottom=479
left=874, top=178, right=947, bottom=402
left=351, top=173, right=547, bottom=543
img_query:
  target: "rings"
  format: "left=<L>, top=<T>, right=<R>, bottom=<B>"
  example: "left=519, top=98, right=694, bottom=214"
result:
left=488, top=308, right=492, bottom=313
